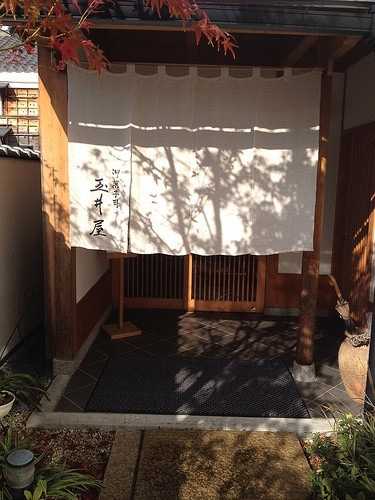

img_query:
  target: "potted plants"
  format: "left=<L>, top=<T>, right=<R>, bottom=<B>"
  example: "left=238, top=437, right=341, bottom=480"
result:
left=0, top=373, right=51, bottom=417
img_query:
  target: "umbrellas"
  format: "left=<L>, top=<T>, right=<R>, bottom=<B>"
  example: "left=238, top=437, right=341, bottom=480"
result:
left=326, top=273, right=359, bottom=336
